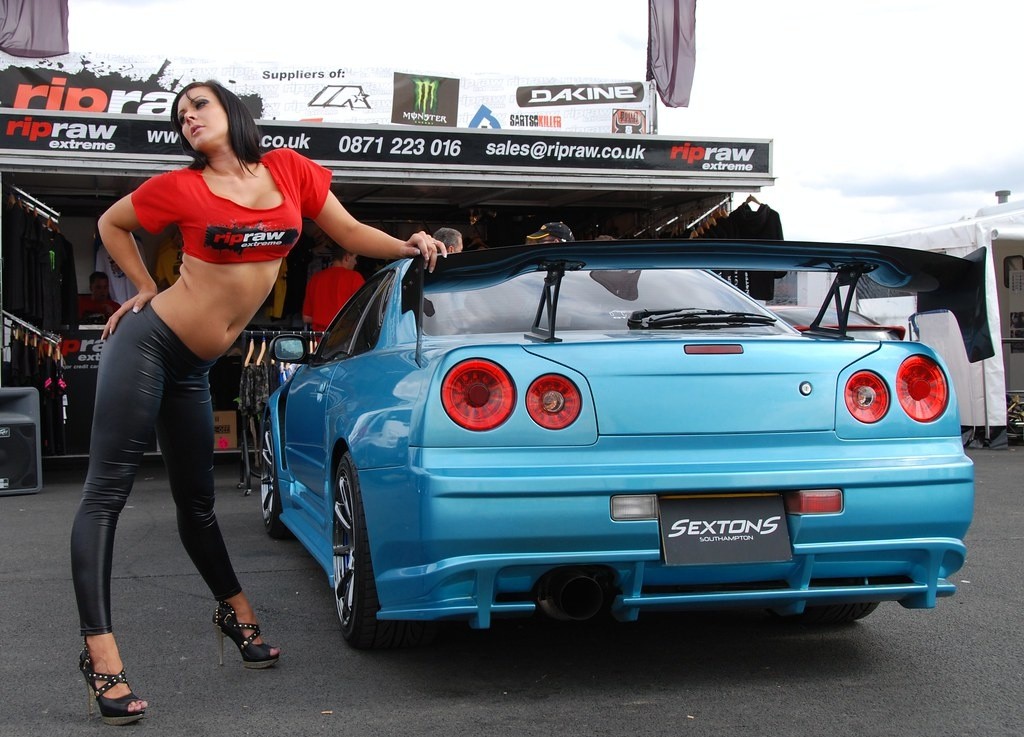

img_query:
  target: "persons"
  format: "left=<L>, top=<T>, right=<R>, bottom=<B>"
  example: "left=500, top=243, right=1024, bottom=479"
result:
left=300, top=242, right=369, bottom=350
left=65, top=80, right=450, bottom=723
left=76, top=271, right=120, bottom=322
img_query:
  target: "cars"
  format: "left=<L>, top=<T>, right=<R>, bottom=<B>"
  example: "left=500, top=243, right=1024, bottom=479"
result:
left=256, top=237, right=996, bottom=652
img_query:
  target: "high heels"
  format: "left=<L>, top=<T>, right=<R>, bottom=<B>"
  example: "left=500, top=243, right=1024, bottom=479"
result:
left=79, top=638, right=145, bottom=726
left=212, top=600, right=279, bottom=669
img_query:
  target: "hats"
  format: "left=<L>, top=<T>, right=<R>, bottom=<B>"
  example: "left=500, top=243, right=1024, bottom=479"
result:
left=526, top=221, right=576, bottom=242
